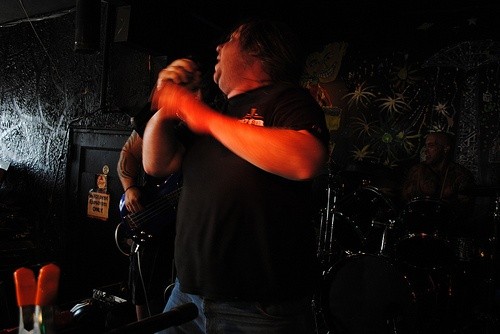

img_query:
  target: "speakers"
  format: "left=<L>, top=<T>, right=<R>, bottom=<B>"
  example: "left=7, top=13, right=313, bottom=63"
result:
left=73, top=0, right=100, bottom=54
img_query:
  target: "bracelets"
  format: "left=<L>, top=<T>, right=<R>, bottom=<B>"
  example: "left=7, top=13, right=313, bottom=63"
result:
left=124, top=185, right=135, bottom=192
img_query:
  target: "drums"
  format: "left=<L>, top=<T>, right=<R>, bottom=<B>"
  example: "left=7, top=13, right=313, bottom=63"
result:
left=352, top=186, right=392, bottom=216
left=404, top=195, right=442, bottom=220
left=361, top=219, right=406, bottom=249
left=312, top=251, right=421, bottom=332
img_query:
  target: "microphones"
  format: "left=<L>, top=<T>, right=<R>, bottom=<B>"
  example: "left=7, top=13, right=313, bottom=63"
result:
left=172, top=53, right=200, bottom=129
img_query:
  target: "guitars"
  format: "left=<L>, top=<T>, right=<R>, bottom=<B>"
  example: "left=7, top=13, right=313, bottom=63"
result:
left=114, top=184, right=179, bottom=257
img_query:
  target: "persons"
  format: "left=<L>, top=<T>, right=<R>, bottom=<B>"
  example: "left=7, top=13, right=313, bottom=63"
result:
left=403, top=133, right=474, bottom=203
left=117, top=73, right=212, bottom=213
left=141, top=16, right=330, bottom=334
